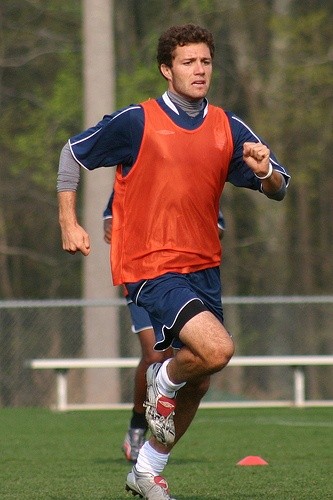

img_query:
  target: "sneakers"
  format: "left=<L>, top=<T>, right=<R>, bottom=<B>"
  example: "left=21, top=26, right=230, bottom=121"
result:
left=122, top=427, right=145, bottom=463
left=125, top=467, right=177, bottom=500
left=144, top=363, right=178, bottom=445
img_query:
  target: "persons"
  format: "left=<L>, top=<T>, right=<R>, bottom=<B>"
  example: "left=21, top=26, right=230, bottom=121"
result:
left=58, top=24, right=291, bottom=500
left=103, top=182, right=225, bottom=464
